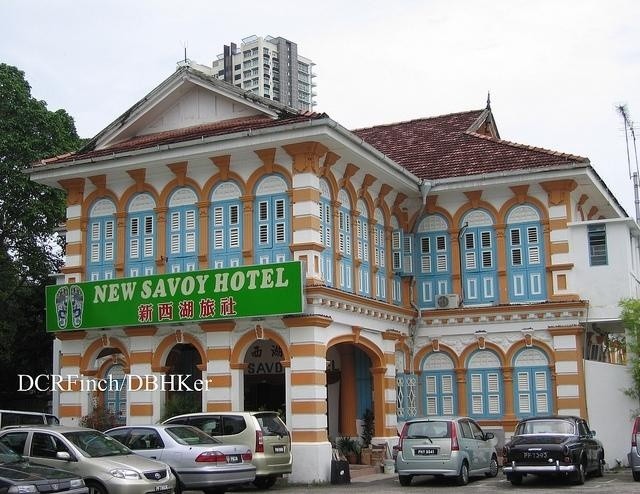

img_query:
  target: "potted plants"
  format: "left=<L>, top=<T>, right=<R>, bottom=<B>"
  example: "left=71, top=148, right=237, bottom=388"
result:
left=337, top=409, right=375, bottom=465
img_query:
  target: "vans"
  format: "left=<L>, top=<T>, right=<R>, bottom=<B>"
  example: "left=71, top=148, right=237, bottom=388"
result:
left=161, top=410, right=293, bottom=489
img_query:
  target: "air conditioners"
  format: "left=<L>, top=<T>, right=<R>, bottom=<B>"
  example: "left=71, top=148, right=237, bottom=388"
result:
left=435, top=294, right=459, bottom=310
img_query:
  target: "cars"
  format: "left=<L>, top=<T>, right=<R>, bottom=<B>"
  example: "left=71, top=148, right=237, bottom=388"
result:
left=630, top=414, right=640, bottom=482
left=0, top=440, right=90, bottom=494
left=83, top=421, right=257, bottom=494
left=502, top=415, right=606, bottom=484
left=0, top=423, right=177, bottom=493
left=395, top=416, right=499, bottom=486
